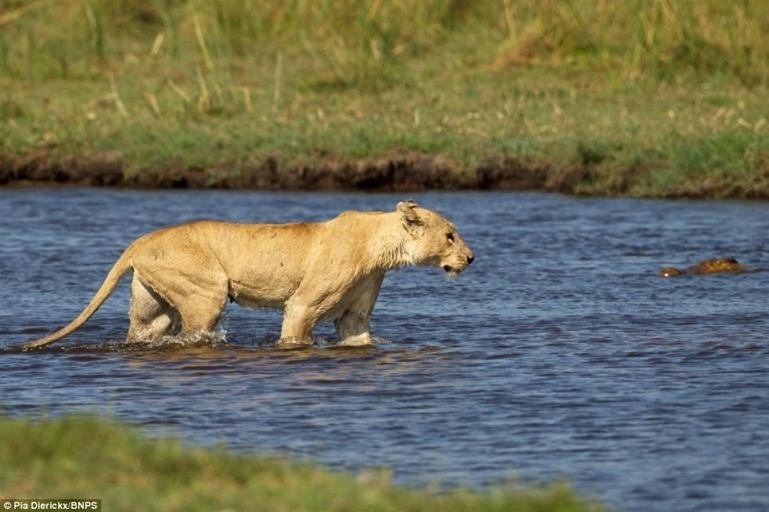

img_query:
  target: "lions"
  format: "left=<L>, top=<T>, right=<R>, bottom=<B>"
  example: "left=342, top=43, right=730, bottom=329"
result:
left=15, top=197, right=475, bottom=348
